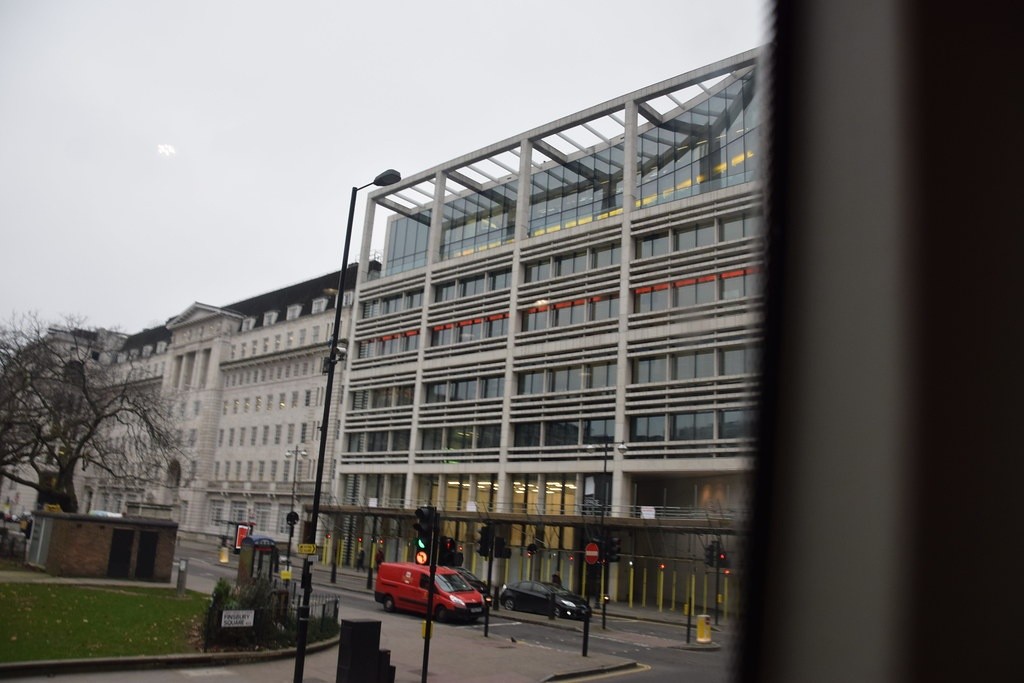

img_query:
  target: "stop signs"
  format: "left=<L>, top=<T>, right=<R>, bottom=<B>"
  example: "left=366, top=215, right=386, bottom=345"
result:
left=584, top=543, right=600, bottom=564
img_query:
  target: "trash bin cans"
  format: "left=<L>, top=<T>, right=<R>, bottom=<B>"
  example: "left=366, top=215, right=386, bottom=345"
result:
left=335, top=618, right=383, bottom=683
left=695, top=614, right=712, bottom=644
left=218, top=535, right=233, bottom=549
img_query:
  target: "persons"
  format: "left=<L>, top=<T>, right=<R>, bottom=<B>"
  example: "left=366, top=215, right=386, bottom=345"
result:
left=25, top=520, right=33, bottom=539
left=553, top=574, right=562, bottom=586
left=375, top=548, right=385, bottom=573
left=356, top=549, right=365, bottom=572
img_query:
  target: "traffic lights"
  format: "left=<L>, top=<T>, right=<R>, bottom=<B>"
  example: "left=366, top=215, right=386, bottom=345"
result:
left=705, top=537, right=731, bottom=570
left=476, top=525, right=490, bottom=556
left=492, top=536, right=513, bottom=561
left=439, top=536, right=457, bottom=567
left=411, top=505, right=437, bottom=567
left=607, top=538, right=622, bottom=566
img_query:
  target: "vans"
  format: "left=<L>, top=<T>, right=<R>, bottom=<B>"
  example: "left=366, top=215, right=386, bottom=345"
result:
left=374, top=560, right=488, bottom=625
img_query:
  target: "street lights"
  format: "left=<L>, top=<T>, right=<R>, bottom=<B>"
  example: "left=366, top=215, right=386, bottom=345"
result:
left=286, top=442, right=309, bottom=564
left=287, top=167, right=402, bottom=683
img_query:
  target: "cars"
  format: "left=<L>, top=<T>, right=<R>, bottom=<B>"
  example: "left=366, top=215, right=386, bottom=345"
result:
left=499, top=580, right=592, bottom=620
left=451, top=565, right=491, bottom=607
